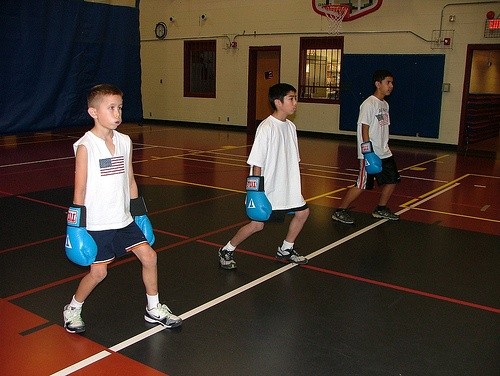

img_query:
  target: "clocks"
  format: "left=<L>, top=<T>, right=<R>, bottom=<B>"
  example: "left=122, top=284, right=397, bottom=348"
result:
left=155, top=22, right=167, bottom=40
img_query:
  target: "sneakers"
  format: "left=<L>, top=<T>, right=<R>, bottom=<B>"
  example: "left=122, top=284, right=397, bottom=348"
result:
left=143, top=303, right=182, bottom=328
left=63, top=303, right=85, bottom=333
left=218, top=247, right=238, bottom=269
left=372, top=207, right=400, bottom=220
left=275, top=246, right=308, bottom=265
left=332, top=210, right=355, bottom=224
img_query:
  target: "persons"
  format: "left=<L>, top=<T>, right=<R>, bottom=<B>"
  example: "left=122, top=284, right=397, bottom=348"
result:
left=219, top=83, right=309, bottom=270
left=332, top=71, right=400, bottom=223
left=64, top=84, right=183, bottom=333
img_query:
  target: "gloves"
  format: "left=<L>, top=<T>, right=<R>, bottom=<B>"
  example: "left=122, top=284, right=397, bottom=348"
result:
left=130, top=196, right=155, bottom=246
left=245, top=176, right=272, bottom=221
left=65, top=204, right=99, bottom=266
left=287, top=212, right=295, bottom=215
left=361, top=141, right=383, bottom=174
